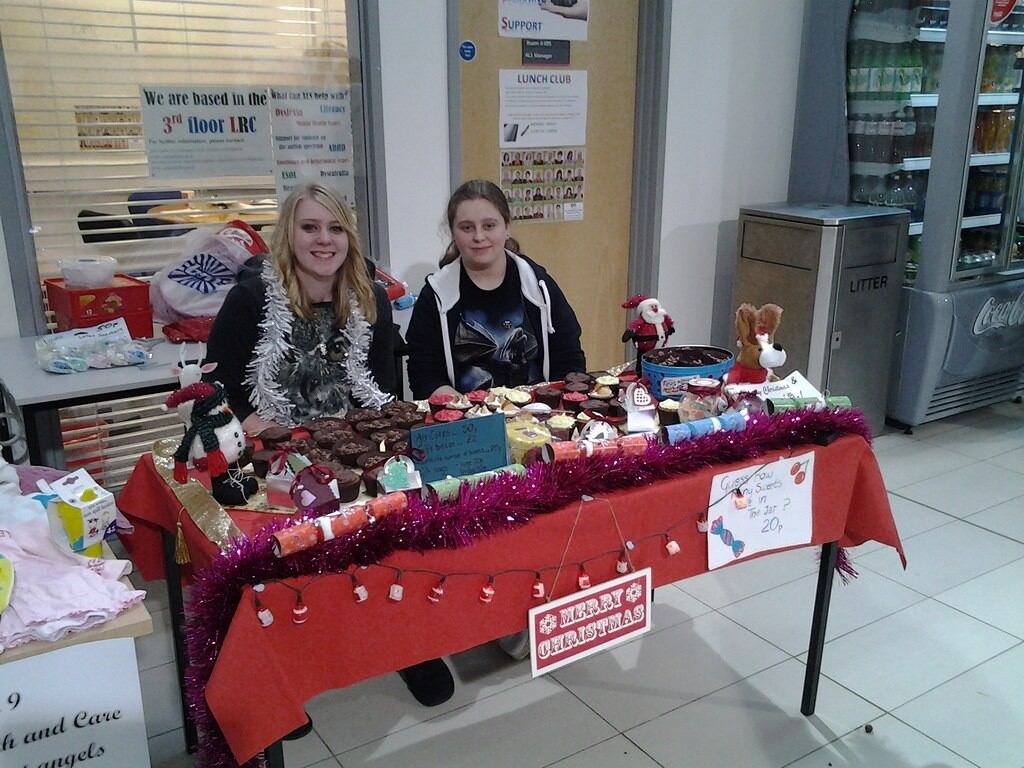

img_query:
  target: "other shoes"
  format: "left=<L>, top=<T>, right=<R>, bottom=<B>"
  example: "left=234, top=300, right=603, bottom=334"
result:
left=398, top=657, right=455, bottom=707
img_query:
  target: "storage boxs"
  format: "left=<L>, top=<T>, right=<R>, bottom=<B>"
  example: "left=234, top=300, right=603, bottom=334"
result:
left=728, top=199, right=912, bottom=439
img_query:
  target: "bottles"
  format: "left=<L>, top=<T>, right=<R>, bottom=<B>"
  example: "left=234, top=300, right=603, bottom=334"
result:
left=844, top=39, right=1022, bottom=279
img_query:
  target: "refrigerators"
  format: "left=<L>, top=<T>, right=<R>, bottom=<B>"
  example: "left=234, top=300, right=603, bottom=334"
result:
left=787, top=1, right=1024, bottom=436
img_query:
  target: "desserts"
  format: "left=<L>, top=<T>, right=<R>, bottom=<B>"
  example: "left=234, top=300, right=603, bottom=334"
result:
left=251, top=369, right=675, bottom=503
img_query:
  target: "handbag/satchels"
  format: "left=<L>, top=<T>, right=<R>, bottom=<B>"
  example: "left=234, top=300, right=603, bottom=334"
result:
left=157, top=235, right=254, bottom=317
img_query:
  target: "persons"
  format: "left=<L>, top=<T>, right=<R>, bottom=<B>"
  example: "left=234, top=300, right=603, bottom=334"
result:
left=406, top=178, right=586, bottom=662
left=196, top=183, right=457, bottom=741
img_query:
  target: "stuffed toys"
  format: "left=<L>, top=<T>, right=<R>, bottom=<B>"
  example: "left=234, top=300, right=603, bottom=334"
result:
left=622, top=295, right=675, bottom=378
left=721, top=303, right=788, bottom=395
left=164, top=380, right=259, bottom=507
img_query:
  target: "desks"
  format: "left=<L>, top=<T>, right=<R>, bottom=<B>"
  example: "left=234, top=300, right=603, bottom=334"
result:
left=0, top=334, right=208, bottom=481
left=141, top=361, right=868, bottom=767
left=0, top=536, right=156, bottom=768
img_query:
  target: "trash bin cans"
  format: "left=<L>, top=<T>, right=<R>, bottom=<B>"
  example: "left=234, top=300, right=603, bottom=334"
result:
left=726, top=200, right=911, bottom=437
